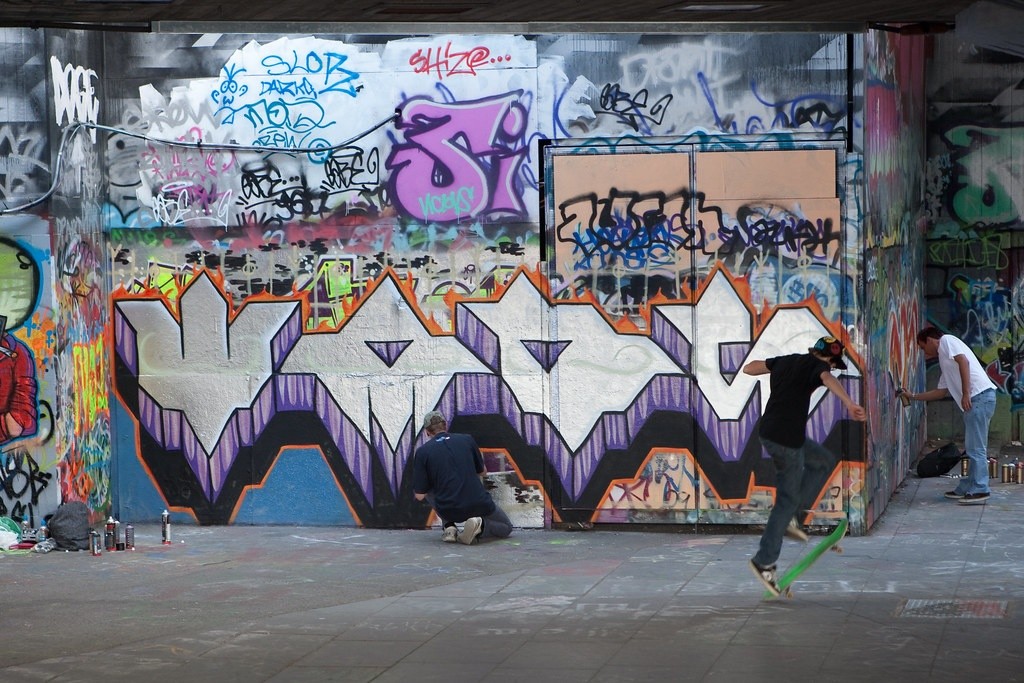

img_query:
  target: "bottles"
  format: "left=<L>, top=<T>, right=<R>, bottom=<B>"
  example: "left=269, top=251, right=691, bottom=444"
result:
left=162, top=510, right=172, bottom=545
left=92, top=532, right=102, bottom=557
left=104, top=524, right=107, bottom=547
left=22, top=514, right=29, bottom=534
left=105, top=516, right=116, bottom=551
left=125, top=523, right=135, bottom=551
left=89, top=528, right=94, bottom=553
left=39, top=520, right=47, bottom=542
left=898, top=388, right=911, bottom=407
left=113, top=517, right=120, bottom=546
left=962, top=457, right=1023, bottom=485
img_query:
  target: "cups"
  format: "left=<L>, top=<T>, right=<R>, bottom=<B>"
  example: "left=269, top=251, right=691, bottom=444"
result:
left=117, top=543, right=125, bottom=550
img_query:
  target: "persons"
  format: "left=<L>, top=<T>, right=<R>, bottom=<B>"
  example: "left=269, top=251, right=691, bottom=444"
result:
left=411, top=410, right=512, bottom=545
left=895, top=327, right=997, bottom=503
left=743, top=336, right=869, bottom=597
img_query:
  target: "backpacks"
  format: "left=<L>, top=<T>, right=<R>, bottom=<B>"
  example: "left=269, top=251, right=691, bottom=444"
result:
left=917, top=442, right=967, bottom=477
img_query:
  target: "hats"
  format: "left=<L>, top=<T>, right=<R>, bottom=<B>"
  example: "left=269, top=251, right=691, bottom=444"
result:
left=814, top=336, right=847, bottom=370
left=424, top=411, right=447, bottom=436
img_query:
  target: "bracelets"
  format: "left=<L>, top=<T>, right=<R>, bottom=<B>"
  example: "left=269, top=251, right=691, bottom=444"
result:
left=912, top=393, right=915, bottom=398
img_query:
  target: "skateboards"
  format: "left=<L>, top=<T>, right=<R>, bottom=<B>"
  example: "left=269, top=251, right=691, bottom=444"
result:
left=763, top=517, right=848, bottom=601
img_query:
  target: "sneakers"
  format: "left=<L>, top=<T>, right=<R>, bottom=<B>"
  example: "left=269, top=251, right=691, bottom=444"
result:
left=945, top=491, right=965, bottom=499
left=749, top=556, right=780, bottom=596
left=457, top=517, right=483, bottom=545
left=440, top=526, right=458, bottom=542
left=958, top=491, right=991, bottom=502
left=785, top=515, right=807, bottom=541
